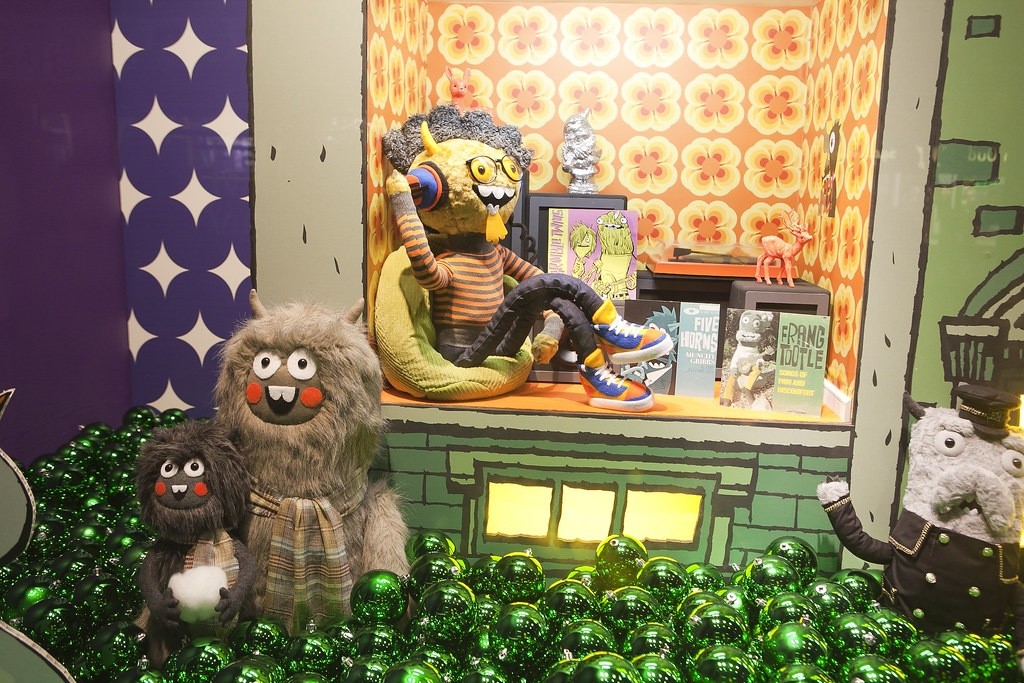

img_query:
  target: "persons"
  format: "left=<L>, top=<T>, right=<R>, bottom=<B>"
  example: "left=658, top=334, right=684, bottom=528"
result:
left=132, top=424, right=261, bottom=670
left=381, top=106, right=675, bottom=411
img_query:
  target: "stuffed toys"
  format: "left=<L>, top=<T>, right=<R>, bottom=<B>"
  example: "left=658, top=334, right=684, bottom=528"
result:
left=813, top=381, right=1024, bottom=636
left=213, top=287, right=415, bottom=634
